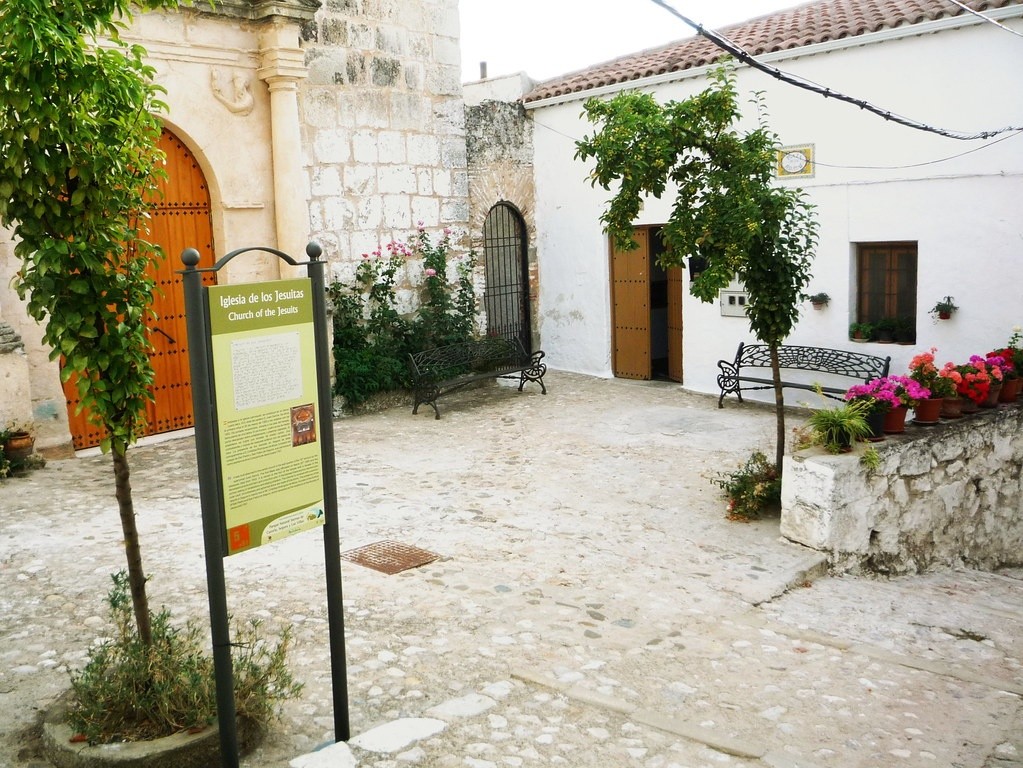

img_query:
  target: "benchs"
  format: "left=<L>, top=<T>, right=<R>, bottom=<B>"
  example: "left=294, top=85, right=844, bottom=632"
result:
left=407, top=335, right=546, bottom=419
left=718, top=342, right=891, bottom=409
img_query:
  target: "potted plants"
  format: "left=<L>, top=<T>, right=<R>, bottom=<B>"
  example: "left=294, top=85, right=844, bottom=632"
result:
left=1010, top=331, right=1023, bottom=393
left=703, top=383, right=877, bottom=510
left=928, top=295, right=960, bottom=326
left=4, top=423, right=35, bottom=459
left=811, top=292, right=830, bottom=311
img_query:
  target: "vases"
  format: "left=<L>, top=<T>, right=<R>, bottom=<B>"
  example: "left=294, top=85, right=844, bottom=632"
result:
left=855, top=380, right=1004, bottom=442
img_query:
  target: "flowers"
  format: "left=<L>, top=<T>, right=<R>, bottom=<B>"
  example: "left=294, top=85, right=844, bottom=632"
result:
left=843, top=346, right=1019, bottom=412
